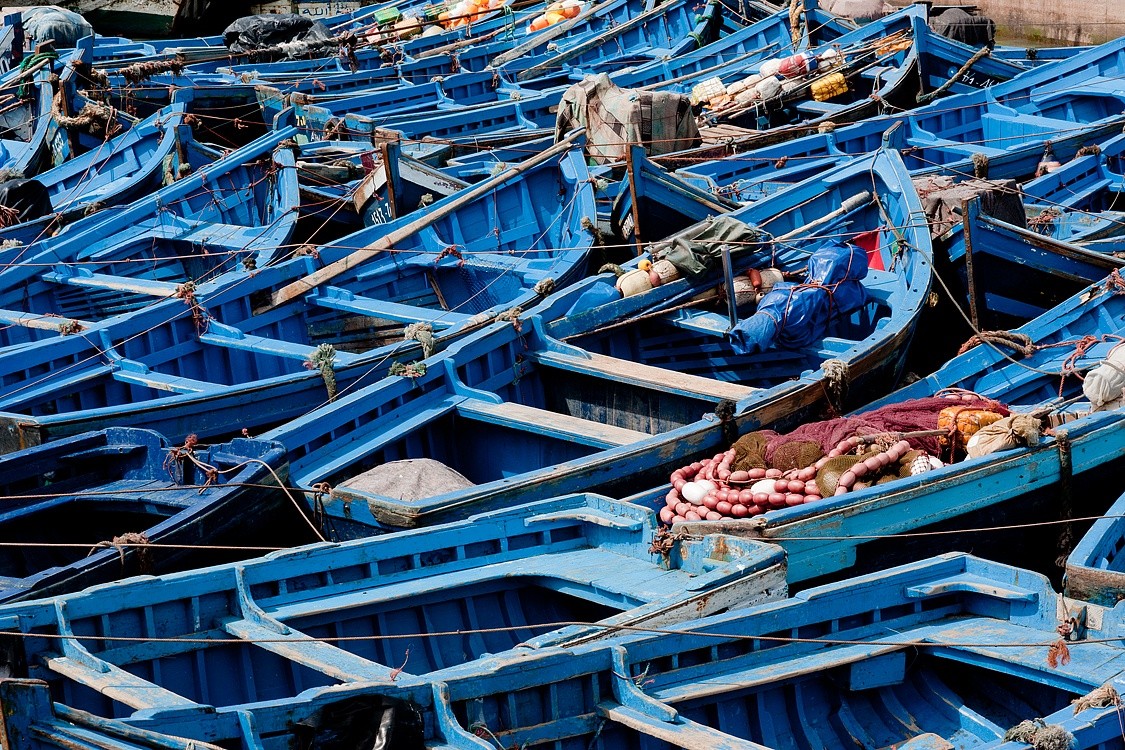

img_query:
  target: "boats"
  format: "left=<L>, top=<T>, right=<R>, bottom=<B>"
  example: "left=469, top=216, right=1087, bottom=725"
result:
left=1, top=487, right=790, bottom=749
left=285, top=121, right=935, bottom=542
left=0, top=0, right=1125, bottom=750
